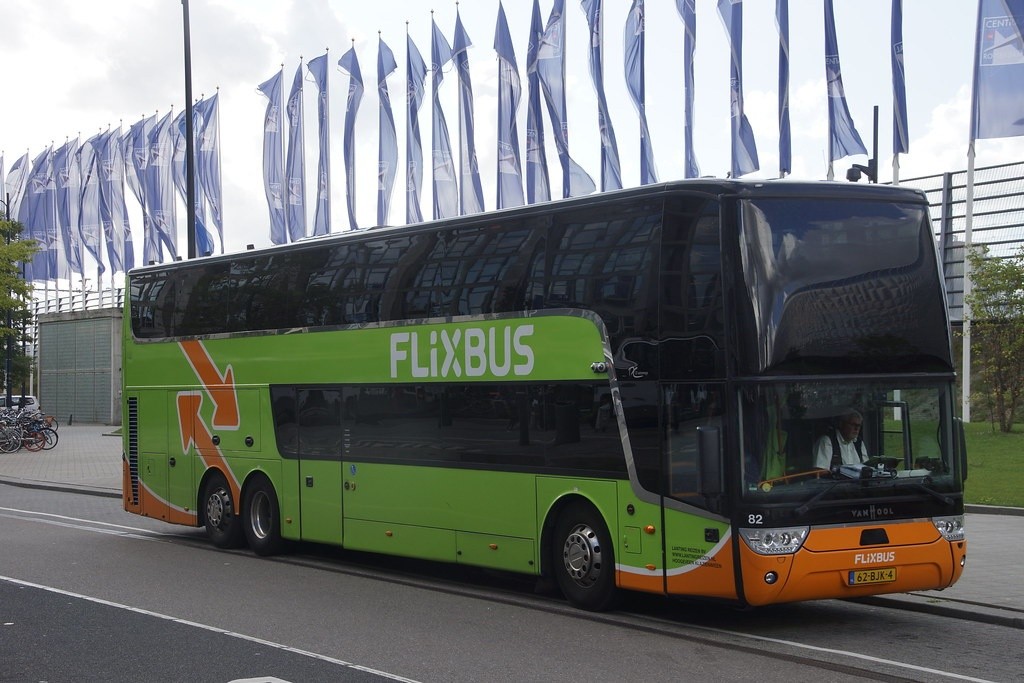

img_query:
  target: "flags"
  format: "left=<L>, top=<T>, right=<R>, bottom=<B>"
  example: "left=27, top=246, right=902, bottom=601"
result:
left=0, top=0, right=1024, bottom=283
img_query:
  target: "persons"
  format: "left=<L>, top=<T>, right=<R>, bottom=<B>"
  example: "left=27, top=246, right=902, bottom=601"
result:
left=814, top=408, right=870, bottom=476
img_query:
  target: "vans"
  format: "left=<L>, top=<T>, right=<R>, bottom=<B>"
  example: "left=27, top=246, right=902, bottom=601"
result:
left=0, top=395, right=41, bottom=418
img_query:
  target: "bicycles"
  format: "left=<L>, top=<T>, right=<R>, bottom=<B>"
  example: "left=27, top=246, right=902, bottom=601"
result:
left=0, top=408, right=59, bottom=453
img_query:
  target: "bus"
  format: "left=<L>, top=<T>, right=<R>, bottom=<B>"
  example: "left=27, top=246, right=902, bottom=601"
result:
left=120, top=178, right=967, bottom=614
